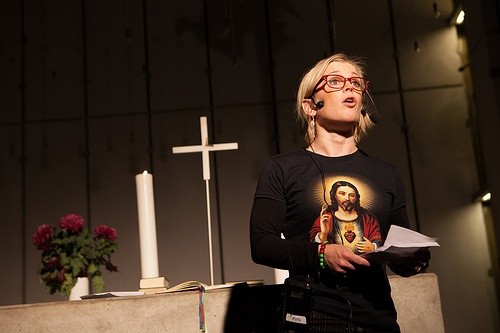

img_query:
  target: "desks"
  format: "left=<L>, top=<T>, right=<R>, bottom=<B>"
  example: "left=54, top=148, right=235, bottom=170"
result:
left=0, top=272, right=445, bottom=333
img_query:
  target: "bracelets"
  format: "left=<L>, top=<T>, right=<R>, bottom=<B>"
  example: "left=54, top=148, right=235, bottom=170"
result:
left=320, top=241, right=328, bottom=270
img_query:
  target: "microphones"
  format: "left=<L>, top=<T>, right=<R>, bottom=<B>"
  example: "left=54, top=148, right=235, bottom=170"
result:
left=312, top=97, right=324, bottom=109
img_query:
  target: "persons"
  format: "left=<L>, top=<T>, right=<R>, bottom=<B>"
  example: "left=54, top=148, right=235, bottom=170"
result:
left=250, top=54, right=430, bottom=332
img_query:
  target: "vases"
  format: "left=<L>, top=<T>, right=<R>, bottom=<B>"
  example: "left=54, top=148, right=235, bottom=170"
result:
left=68, top=276, right=89, bottom=301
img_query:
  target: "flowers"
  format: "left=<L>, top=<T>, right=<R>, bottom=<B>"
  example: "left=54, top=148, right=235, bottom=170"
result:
left=31, top=214, right=120, bottom=298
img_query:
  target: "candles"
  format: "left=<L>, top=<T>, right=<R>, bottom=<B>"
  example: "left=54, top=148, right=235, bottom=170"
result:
left=135, top=169, right=160, bottom=278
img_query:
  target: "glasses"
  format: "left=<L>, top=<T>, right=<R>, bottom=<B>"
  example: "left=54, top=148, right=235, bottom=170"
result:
left=313, top=75, right=370, bottom=92
